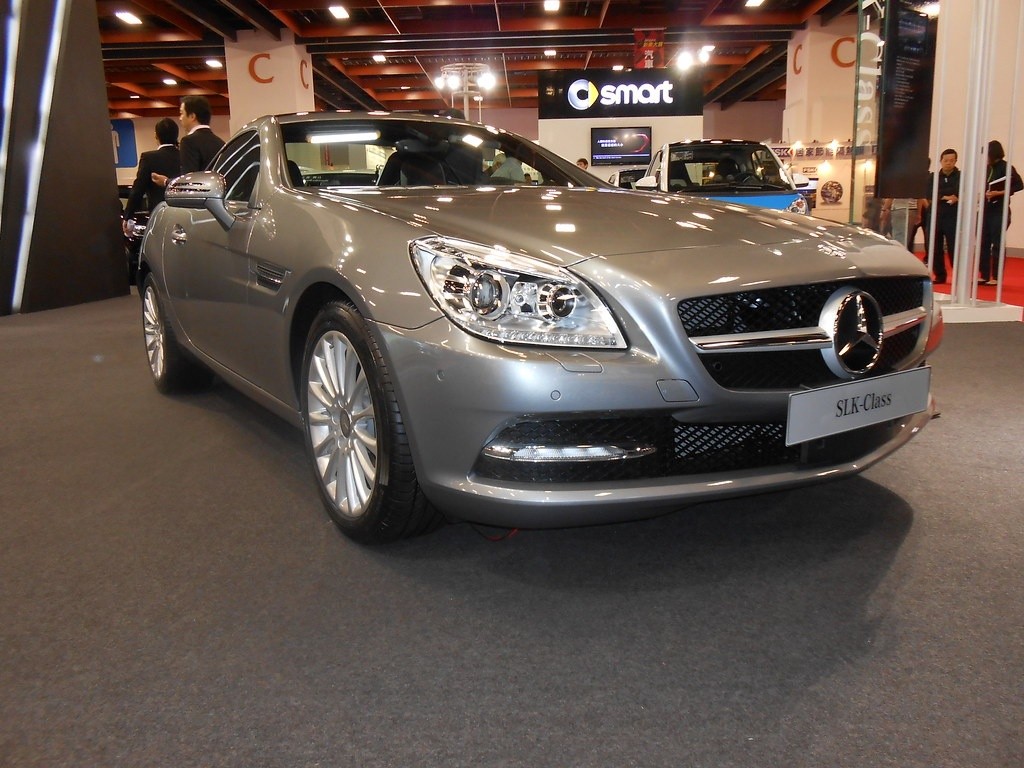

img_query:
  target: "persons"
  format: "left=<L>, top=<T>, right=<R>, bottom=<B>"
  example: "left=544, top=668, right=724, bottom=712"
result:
left=483, top=153, right=555, bottom=186
left=576, top=158, right=589, bottom=170
left=879, top=148, right=961, bottom=283
left=976, top=139, right=1024, bottom=286
left=118, top=93, right=228, bottom=238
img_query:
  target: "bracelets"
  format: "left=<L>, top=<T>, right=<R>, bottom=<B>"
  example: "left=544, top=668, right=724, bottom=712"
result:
left=163, top=178, right=167, bottom=187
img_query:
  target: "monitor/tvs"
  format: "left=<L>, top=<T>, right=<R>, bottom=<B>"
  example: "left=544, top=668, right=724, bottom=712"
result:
left=591, top=126, right=652, bottom=166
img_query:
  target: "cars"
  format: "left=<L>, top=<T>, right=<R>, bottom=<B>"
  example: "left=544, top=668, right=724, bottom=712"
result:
left=634, top=139, right=809, bottom=216
left=135, top=110, right=942, bottom=548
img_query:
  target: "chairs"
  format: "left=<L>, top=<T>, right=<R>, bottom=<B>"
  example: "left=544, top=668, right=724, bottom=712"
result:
left=669, top=161, right=692, bottom=186
left=715, top=157, right=741, bottom=175
left=287, top=160, right=304, bottom=186
left=399, top=153, right=447, bottom=187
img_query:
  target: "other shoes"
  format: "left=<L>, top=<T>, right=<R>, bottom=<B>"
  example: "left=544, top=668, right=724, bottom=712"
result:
left=984, top=280, right=998, bottom=286
left=978, top=278, right=989, bottom=284
left=932, top=279, right=946, bottom=285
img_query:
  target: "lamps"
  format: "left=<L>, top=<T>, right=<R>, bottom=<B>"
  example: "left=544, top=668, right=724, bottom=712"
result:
left=305, top=129, right=381, bottom=143
left=433, top=61, right=496, bottom=124
